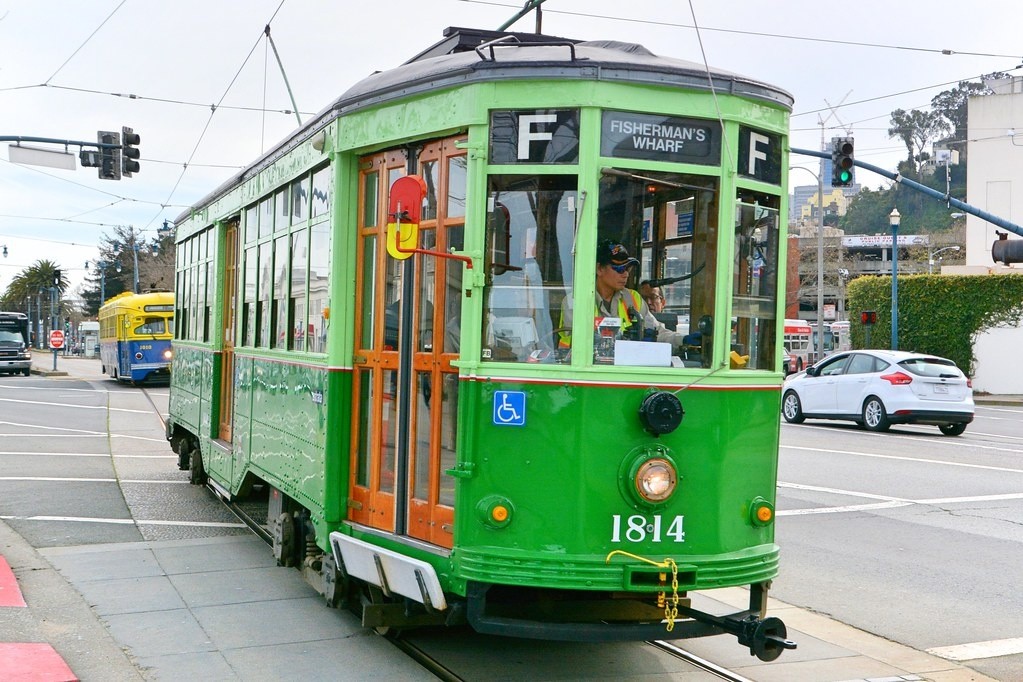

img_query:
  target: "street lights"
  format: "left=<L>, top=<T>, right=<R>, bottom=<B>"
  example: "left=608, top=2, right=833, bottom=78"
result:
left=85, top=261, right=122, bottom=305
left=113, top=242, right=160, bottom=293
left=39, top=286, right=55, bottom=352
left=890, top=208, right=901, bottom=348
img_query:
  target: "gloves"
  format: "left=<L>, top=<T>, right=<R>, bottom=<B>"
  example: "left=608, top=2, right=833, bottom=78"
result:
left=683, top=331, right=703, bottom=345
left=643, top=327, right=659, bottom=342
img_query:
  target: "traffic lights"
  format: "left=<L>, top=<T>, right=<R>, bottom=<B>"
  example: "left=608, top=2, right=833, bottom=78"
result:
left=860, top=313, right=876, bottom=323
left=72, top=336, right=76, bottom=341
left=832, top=137, right=854, bottom=188
left=120, top=126, right=140, bottom=178
left=65, top=317, right=69, bottom=327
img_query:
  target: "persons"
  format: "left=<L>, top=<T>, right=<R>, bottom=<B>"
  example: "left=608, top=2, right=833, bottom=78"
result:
left=637, top=279, right=666, bottom=314
left=558, top=239, right=701, bottom=349
left=387, top=295, right=537, bottom=475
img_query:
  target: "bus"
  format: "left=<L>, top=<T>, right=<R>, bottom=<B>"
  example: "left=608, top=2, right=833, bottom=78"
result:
left=98, top=291, right=175, bottom=388
left=730, top=318, right=815, bottom=378
left=810, top=321, right=853, bottom=362
left=164, top=33, right=797, bottom=665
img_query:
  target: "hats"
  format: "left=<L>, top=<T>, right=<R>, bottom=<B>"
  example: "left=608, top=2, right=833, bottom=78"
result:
left=596, top=239, right=640, bottom=268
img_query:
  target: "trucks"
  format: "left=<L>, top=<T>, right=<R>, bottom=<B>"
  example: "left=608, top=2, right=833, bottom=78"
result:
left=0, top=312, right=32, bottom=377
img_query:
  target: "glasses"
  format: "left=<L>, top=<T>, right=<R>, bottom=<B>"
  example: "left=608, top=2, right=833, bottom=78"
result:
left=643, top=294, right=663, bottom=299
left=608, top=263, right=633, bottom=274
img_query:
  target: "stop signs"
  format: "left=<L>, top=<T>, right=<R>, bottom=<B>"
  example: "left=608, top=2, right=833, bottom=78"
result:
left=49, top=330, right=65, bottom=348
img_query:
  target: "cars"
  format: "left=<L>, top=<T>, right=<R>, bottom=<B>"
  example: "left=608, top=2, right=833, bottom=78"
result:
left=63, top=342, right=100, bottom=353
left=781, top=349, right=976, bottom=436
